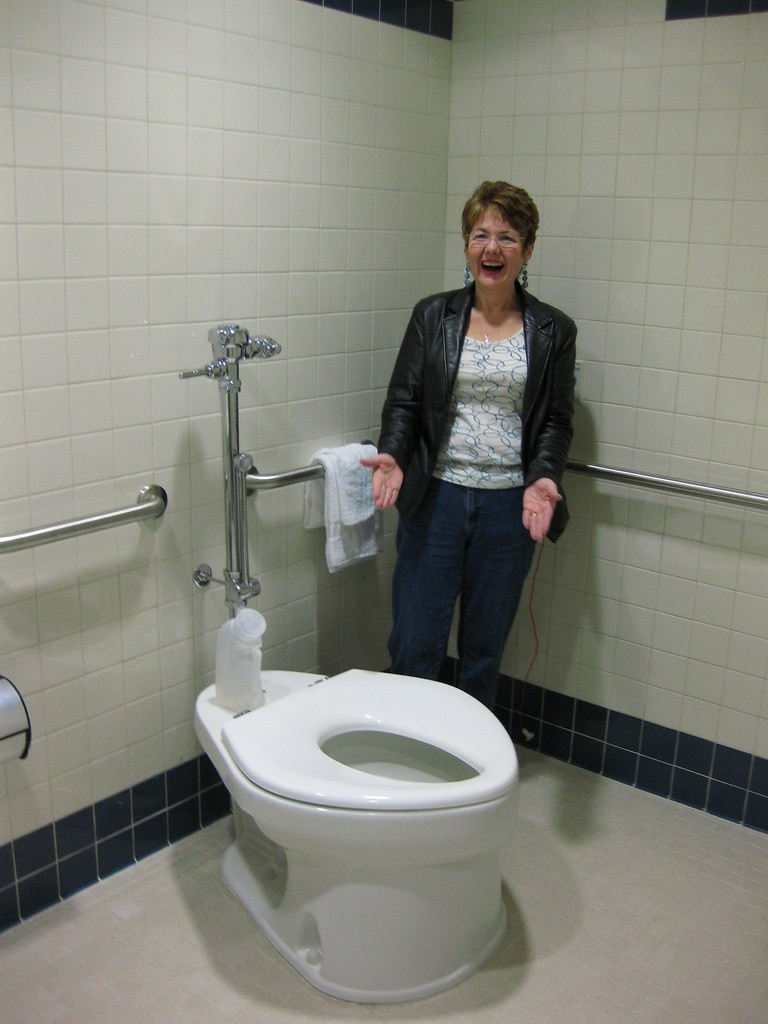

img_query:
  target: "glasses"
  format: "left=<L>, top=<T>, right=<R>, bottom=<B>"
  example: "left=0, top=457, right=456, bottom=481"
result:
left=467, top=234, right=525, bottom=247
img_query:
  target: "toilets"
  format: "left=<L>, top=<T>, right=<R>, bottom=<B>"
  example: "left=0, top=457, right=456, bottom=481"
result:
left=192, top=666, right=521, bottom=1005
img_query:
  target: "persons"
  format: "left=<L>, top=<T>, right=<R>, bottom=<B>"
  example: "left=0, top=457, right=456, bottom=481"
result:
left=356, top=177, right=579, bottom=727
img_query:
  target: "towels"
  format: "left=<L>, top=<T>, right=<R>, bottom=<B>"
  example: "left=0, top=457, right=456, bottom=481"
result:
left=307, top=439, right=382, bottom=574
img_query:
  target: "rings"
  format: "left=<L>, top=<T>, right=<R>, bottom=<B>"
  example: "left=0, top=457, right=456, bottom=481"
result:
left=530, top=513, right=537, bottom=516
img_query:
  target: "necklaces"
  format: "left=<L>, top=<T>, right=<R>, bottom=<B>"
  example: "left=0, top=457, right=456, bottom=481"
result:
left=476, top=304, right=511, bottom=343
